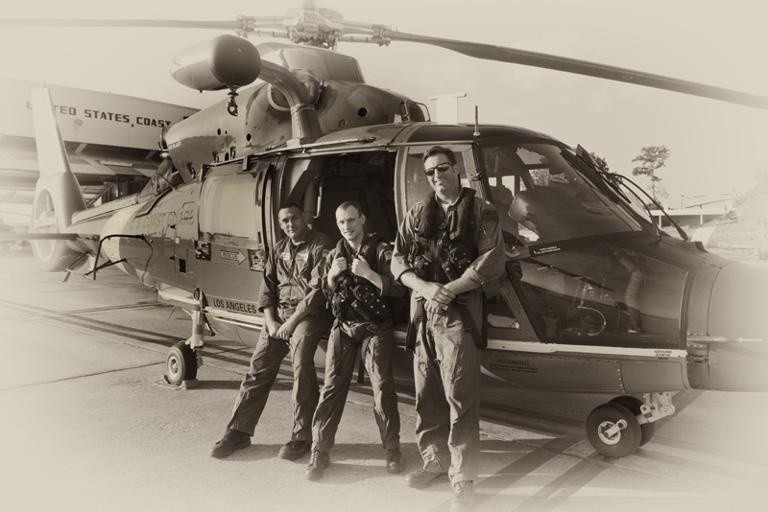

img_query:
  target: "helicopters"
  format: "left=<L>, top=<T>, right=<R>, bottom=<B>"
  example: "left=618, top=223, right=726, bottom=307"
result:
left=0, top=1, right=768, bottom=460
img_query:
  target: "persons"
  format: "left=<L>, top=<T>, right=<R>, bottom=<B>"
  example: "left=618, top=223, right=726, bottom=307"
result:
left=210, top=202, right=334, bottom=461
left=389, top=145, right=507, bottom=512
left=302, top=200, right=409, bottom=482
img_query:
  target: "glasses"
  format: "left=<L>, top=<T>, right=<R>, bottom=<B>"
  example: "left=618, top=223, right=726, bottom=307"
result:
left=422, top=161, right=455, bottom=176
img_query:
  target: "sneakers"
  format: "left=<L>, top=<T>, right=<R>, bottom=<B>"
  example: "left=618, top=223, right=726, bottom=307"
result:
left=401, top=468, right=449, bottom=491
left=450, top=478, right=479, bottom=512
left=303, top=448, right=330, bottom=483
left=382, top=444, right=404, bottom=474
left=210, top=427, right=253, bottom=459
left=277, top=436, right=314, bottom=462
